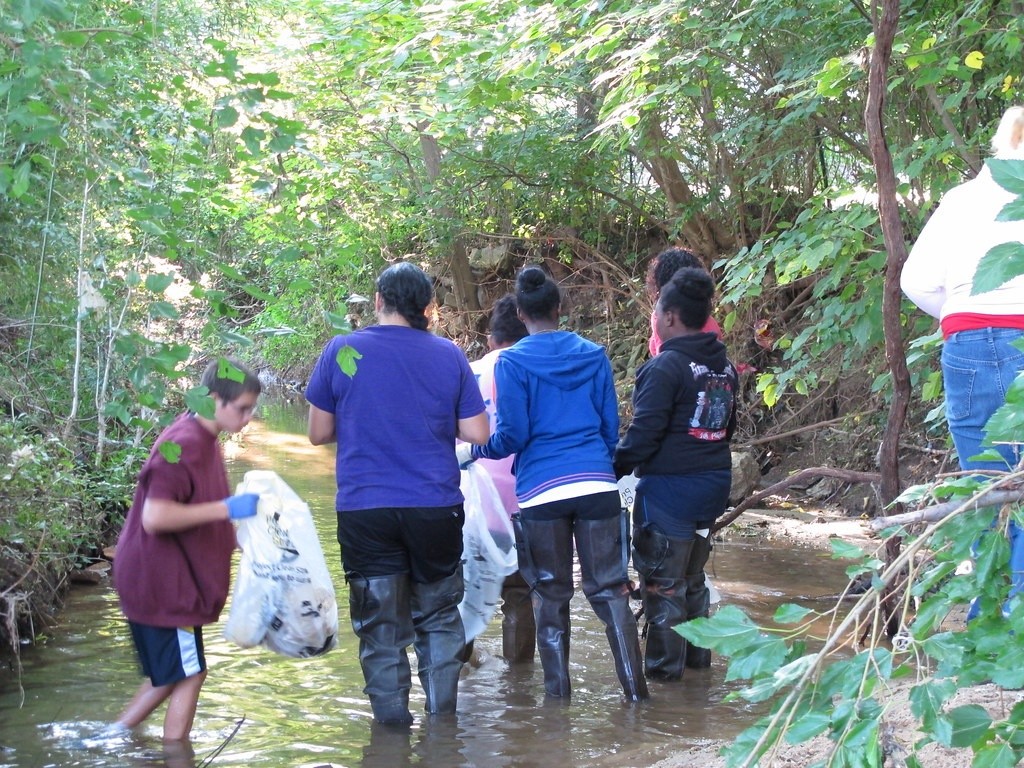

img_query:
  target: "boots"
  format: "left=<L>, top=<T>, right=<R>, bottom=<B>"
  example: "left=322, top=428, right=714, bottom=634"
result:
left=511, top=511, right=574, bottom=698
left=409, top=559, right=468, bottom=718
left=346, top=569, right=415, bottom=725
left=501, top=570, right=536, bottom=664
left=576, top=508, right=649, bottom=702
left=686, top=528, right=713, bottom=672
left=634, top=522, right=696, bottom=685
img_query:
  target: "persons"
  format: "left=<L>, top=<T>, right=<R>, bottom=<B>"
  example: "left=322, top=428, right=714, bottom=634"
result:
left=457, top=294, right=536, bottom=664
left=455, top=268, right=650, bottom=701
left=612, top=267, right=737, bottom=681
left=646, top=247, right=721, bottom=357
left=901, top=106, right=1024, bottom=689
left=106, top=356, right=262, bottom=738
left=308, top=263, right=489, bottom=720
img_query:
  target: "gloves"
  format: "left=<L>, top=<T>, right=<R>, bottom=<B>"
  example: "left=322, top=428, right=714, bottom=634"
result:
left=456, top=442, right=476, bottom=470
left=225, top=493, right=259, bottom=519
